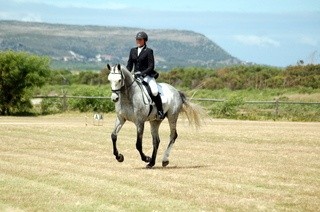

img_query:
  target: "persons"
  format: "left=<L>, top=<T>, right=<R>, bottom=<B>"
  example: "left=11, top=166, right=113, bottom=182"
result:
left=126, top=31, right=164, bottom=120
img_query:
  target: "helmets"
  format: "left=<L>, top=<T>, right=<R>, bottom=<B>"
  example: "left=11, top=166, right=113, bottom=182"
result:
left=136, top=32, right=148, bottom=41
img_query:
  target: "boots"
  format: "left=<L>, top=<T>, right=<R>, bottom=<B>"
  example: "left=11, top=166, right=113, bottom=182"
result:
left=155, top=98, right=165, bottom=119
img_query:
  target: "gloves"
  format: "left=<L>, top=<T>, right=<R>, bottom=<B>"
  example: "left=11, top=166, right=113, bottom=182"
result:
left=134, top=71, right=142, bottom=77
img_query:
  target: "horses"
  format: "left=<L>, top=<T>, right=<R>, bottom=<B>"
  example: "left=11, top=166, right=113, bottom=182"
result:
left=106, top=63, right=214, bottom=168
